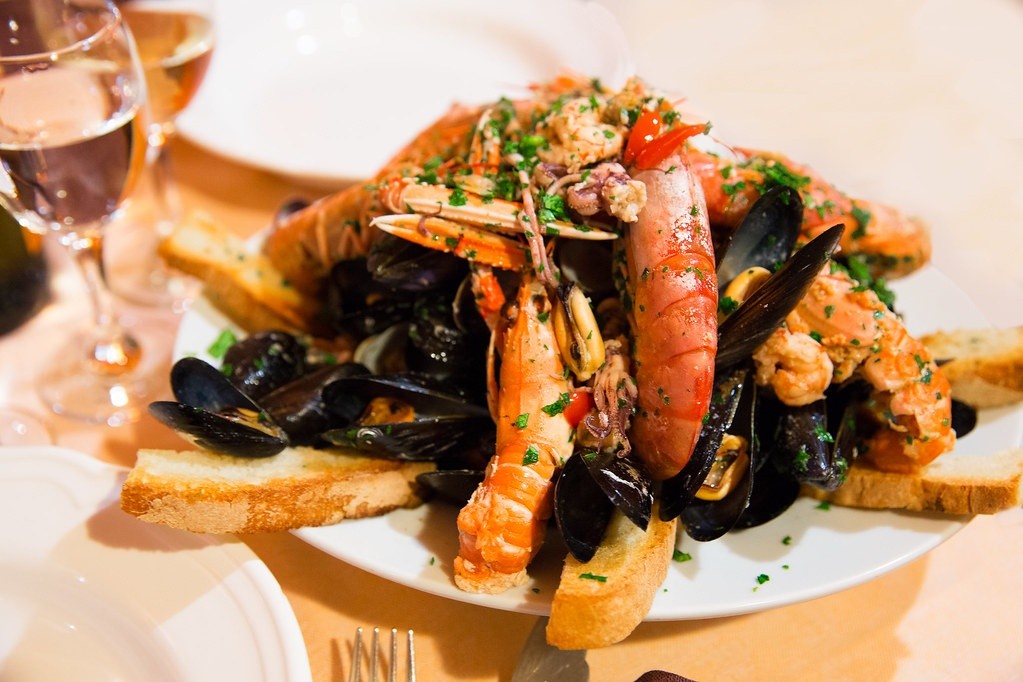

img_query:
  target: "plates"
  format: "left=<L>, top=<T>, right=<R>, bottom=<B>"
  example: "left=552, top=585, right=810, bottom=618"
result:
left=171, top=220, right=1023, bottom=621
left=0, top=440, right=313, bottom=682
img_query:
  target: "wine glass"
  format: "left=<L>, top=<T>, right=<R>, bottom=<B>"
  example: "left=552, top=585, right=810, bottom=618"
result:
left=0, top=1, right=174, bottom=424
left=63, top=0, right=239, bottom=298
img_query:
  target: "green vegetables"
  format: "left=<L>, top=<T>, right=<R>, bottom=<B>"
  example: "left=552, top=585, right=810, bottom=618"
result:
left=210, top=83, right=941, bottom=588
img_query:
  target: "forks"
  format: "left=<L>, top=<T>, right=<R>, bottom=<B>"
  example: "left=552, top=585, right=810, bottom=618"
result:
left=346, top=627, right=417, bottom=682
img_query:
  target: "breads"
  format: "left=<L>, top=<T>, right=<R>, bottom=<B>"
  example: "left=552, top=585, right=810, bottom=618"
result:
left=120, top=443, right=437, bottom=534
left=155, top=209, right=322, bottom=338
left=798, top=443, right=1023, bottom=517
left=916, top=325, right=1023, bottom=407
left=547, top=501, right=677, bottom=653
